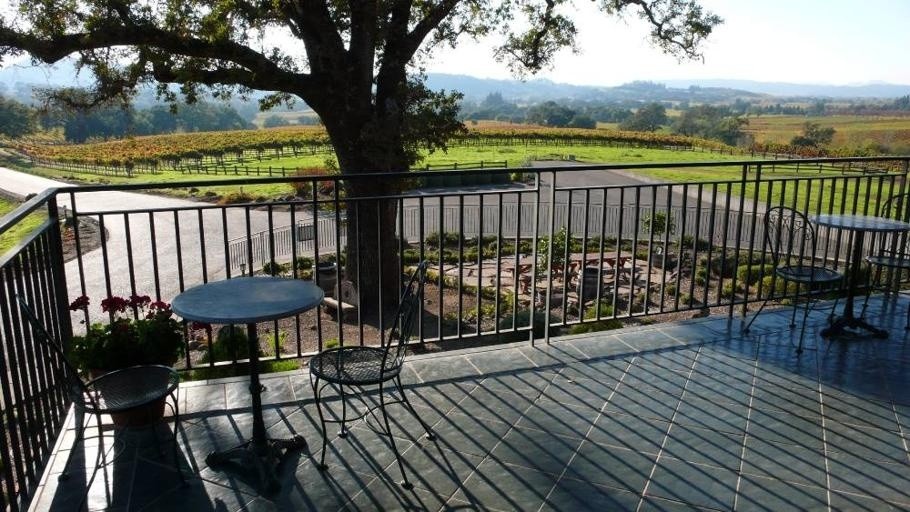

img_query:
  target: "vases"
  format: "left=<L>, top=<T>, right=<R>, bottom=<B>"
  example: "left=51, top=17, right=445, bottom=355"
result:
left=91, top=365, right=166, bottom=430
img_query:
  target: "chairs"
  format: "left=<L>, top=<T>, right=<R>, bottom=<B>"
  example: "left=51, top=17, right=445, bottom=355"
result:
left=308, top=259, right=434, bottom=489
left=862, top=192, right=910, bottom=330
left=12, top=293, right=190, bottom=507
left=742, top=206, right=844, bottom=353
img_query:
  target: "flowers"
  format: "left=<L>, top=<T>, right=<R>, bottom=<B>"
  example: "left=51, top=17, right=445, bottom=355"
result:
left=69, top=295, right=210, bottom=373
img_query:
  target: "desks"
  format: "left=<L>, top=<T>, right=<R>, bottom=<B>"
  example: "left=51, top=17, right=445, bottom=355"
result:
left=809, top=212, right=910, bottom=338
left=172, top=273, right=325, bottom=497
